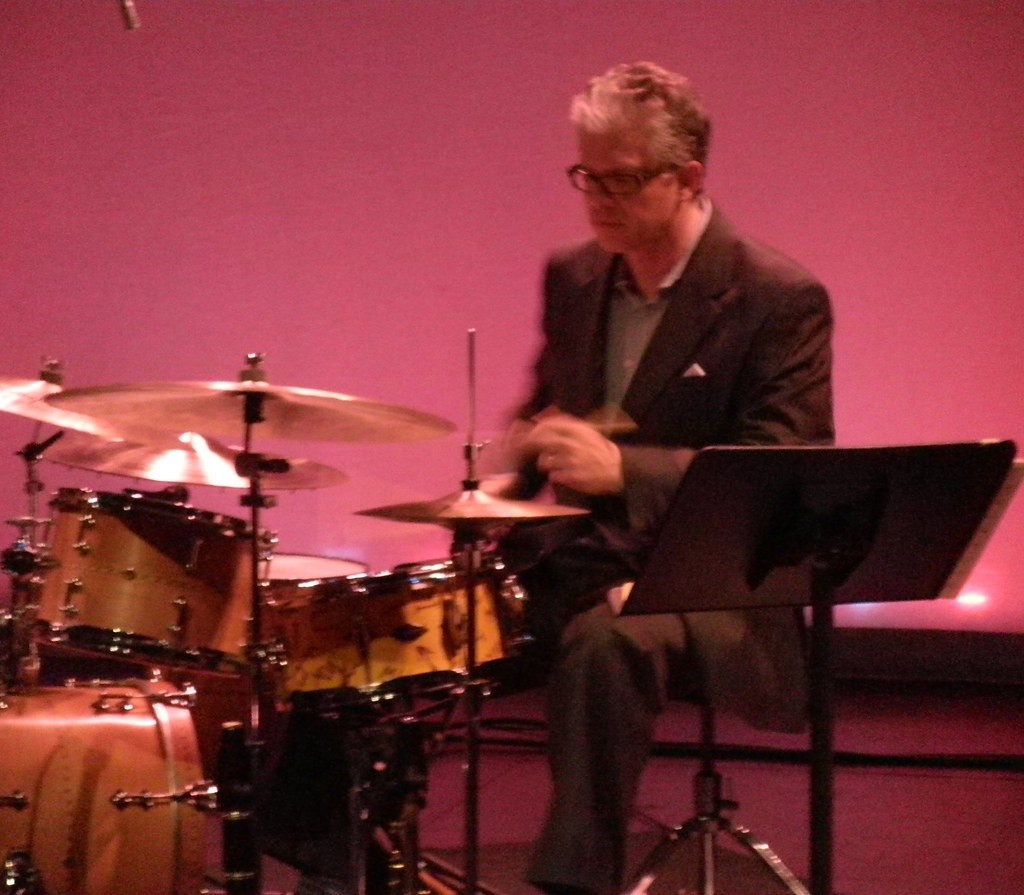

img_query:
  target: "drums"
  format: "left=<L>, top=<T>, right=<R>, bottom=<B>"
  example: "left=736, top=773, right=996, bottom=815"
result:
left=345, top=555, right=527, bottom=705
left=239, top=550, right=373, bottom=697
left=26, top=485, right=277, bottom=680
left=0, top=676, right=228, bottom=895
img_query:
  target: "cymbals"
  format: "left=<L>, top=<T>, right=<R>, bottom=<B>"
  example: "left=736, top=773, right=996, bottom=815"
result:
left=41, top=430, right=354, bottom=492
left=41, top=379, right=460, bottom=445
left=0, top=373, right=198, bottom=454
left=348, top=489, right=592, bottom=534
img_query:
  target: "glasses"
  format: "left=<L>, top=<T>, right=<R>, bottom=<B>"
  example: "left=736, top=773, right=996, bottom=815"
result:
left=567, top=162, right=680, bottom=197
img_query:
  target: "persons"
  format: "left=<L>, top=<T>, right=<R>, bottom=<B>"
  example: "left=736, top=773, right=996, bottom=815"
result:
left=259, top=61, right=836, bottom=895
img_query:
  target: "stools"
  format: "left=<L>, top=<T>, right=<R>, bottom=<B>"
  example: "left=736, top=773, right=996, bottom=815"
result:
left=621, top=686, right=813, bottom=895
